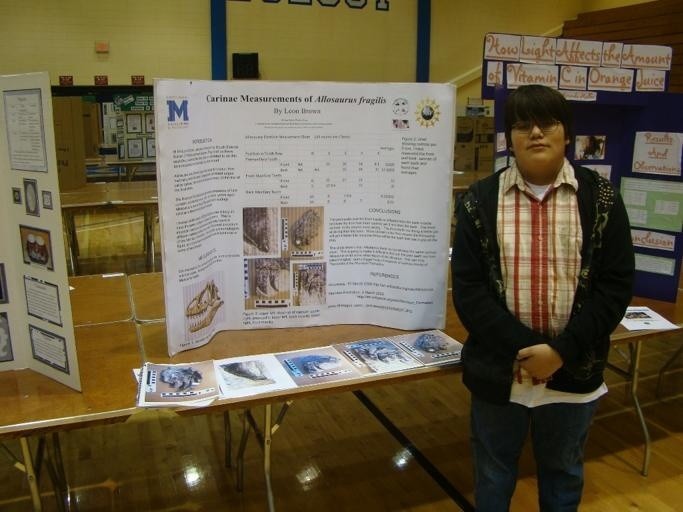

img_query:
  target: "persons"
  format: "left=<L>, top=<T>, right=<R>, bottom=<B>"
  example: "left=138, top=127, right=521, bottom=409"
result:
left=451, top=85, right=635, bottom=512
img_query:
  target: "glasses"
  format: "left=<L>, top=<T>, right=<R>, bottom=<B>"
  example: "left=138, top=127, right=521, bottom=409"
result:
left=510, top=116, right=561, bottom=135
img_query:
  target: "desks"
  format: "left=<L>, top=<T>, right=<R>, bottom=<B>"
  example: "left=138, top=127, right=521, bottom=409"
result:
left=0, top=256, right=682, bottom=510
left=58, top=154, right=160, bottom=279
